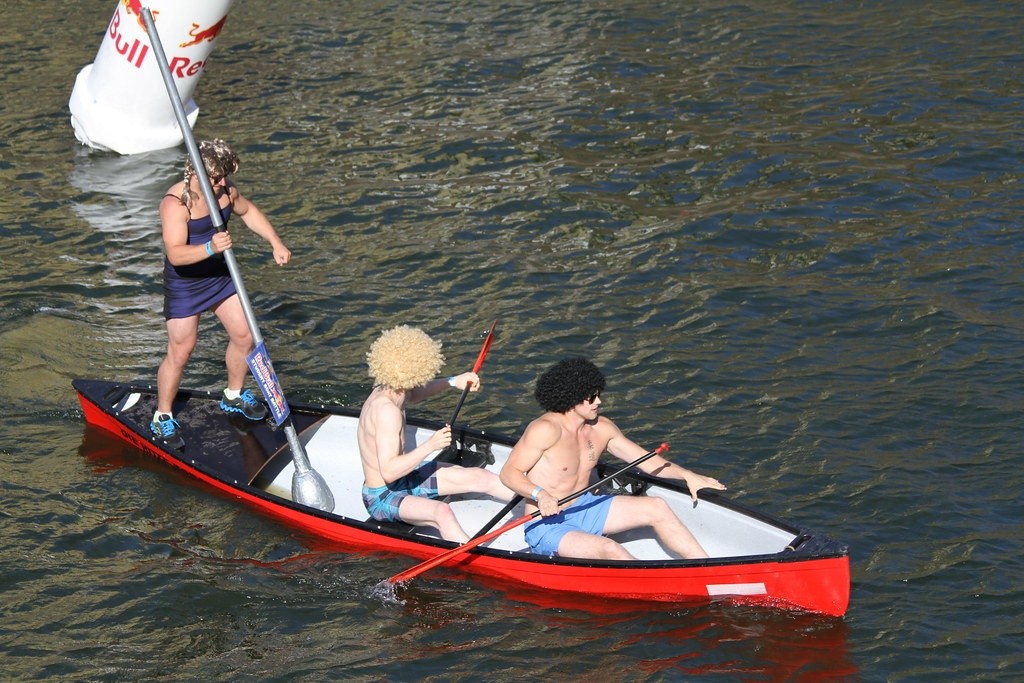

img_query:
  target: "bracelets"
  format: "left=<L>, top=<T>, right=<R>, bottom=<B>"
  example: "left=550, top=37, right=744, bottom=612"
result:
left=449, top=377, right=455, bottom=386
left=531, top=486, right=543, bottom=501
left=206, top=241, right=214, bottom=256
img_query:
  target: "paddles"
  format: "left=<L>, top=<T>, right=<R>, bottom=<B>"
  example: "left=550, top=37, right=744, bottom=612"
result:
left=446, top=319, right=497, bottom=425
left=390, top=442, right=668, bottom=581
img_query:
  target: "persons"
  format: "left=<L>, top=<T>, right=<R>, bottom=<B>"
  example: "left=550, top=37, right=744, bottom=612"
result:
left=356, top=324, right=518, bottom=543
left=150, top=136, right=292, bottom=448
left=499, top=358, right=727, bottom=560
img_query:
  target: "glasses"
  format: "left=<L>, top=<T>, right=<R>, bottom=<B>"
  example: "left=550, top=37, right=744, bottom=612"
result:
left=585, top=390, right=602, bottom=404
left=208, top=175, right=225, bottom=184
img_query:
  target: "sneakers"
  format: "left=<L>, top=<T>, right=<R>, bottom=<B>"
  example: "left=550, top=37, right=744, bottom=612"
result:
left=219, top=389, right=268, bottom=420
left=150, top=415, right=185, bottom=450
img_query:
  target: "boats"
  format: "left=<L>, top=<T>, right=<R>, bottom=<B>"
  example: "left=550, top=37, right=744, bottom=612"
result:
left=72, top=377, right=851, bottom=618
